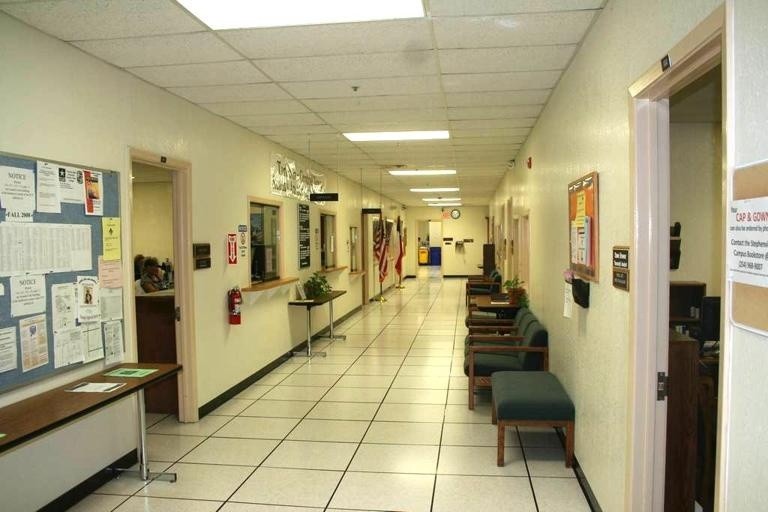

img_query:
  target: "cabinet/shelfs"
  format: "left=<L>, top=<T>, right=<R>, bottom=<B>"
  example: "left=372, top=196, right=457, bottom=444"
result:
left=663, top=278, right=721, bottom=512
left=483, top=244, right=495, bottom=275
left=135, top=297, right=178, bottom=414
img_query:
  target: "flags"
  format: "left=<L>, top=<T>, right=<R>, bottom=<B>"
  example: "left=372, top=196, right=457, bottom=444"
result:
left=392, top=216, right=408, bottom=277
left=373, top=212, right=391, bottom=283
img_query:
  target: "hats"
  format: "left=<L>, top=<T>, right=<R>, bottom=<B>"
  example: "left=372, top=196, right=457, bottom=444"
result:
left=145, top=260, right=161, bottom=267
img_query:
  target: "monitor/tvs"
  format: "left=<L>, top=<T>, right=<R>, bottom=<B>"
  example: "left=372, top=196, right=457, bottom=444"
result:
left=700, top=296, right=720, bottom=340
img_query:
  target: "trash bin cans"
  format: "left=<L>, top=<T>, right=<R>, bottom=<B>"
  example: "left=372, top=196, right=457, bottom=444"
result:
left=430, top=247, right=440, bottom=265
left=419, top=247, right=428, bottom=264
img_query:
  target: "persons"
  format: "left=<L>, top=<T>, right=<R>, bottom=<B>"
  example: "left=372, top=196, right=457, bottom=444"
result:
left=133, top=257, right=168, bottom=296
left=133, top=255, right=147, bottom=281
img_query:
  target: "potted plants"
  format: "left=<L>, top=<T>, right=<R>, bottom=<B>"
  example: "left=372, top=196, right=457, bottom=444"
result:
left=303, top=271, right=332, bottom=299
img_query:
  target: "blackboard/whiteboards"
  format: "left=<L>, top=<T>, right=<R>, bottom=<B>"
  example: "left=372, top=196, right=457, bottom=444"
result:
left=0, top=152, right=125, bottom=394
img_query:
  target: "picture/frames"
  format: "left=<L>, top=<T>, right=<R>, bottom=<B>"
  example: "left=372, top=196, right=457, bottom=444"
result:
left=567, top=171, right=600, bottom=283
left=451, top=209, right=460, bottom=219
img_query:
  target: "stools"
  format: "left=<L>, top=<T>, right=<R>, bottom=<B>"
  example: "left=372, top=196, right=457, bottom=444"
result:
left=490, top=371, right=575, bottom=468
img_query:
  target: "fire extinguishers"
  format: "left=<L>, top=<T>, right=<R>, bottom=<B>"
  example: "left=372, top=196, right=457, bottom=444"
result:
left=228, top=288, right=242, bottom=324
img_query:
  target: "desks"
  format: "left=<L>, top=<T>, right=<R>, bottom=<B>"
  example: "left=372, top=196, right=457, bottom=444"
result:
left=288, top=290, right=347, bottom=358
left=0, top=363, right=182, bottom=484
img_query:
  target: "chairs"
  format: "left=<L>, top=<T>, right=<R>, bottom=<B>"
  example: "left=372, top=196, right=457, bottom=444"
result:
left=464, top=269, right=549, bottom=410
